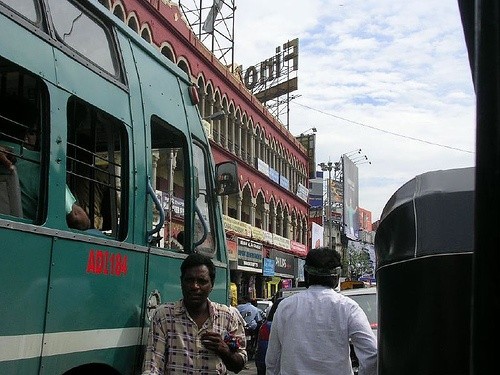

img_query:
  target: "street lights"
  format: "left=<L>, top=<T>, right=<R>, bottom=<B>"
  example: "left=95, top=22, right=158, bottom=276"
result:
left=319, top=156, right=341, bottom=248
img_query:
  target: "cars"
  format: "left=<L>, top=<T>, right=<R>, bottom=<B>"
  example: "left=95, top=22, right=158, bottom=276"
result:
left=255, top=287, right=378, bottom=375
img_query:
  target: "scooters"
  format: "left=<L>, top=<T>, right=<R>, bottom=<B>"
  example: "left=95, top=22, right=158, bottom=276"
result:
left=245, top=317, right=266, bottom=360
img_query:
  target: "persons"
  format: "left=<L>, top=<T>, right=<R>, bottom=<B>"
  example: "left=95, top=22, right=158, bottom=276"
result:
left=346, top=197, right=358, bottom=236
left=249, top=297, right=263, bottom=316
left=236, top=295, right=263, bottom=334
left=66, top=138, right=104, bottom=231
left=142, top=253, right=248, bottom=375
left=12, top=112, right=90, bottom=232
left=264, top=247, right=378, bottom=375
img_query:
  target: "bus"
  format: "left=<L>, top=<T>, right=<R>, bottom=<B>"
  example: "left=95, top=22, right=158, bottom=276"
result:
left=0, top=0, right=240, bottom=375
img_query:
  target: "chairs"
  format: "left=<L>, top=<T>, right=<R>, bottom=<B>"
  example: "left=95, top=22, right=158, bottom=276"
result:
left=0, top=169, right=24, bottom=218
